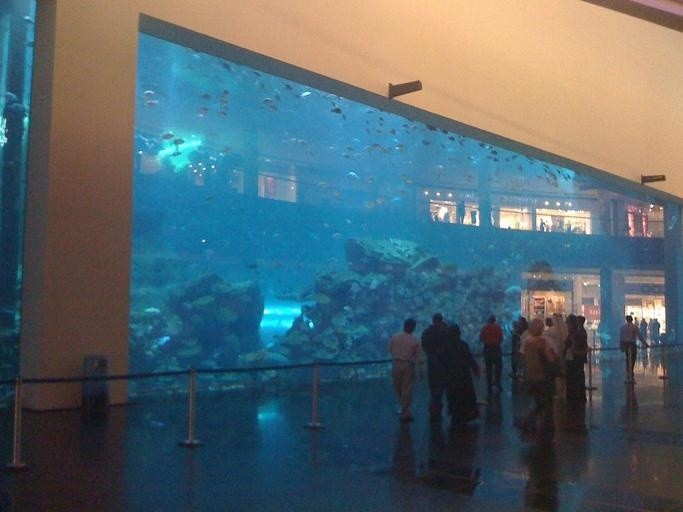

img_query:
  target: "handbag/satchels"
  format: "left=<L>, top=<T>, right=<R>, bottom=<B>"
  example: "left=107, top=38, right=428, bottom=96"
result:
left=539, top=347, right=564, bottom=380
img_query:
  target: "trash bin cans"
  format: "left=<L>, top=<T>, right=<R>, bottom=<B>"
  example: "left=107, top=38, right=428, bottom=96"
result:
left=80, top=354, right=108, bottom=421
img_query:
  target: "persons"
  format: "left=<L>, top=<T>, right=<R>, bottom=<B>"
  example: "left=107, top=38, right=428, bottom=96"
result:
left=432, top=200, right=572, bottom=233
left=421, top=312, right=588, bottom=425
left=386, top=317, right=425, bottom=423
left=621, top=314, right=651, bottom=384
left=633, top=316, right=661, bottom=341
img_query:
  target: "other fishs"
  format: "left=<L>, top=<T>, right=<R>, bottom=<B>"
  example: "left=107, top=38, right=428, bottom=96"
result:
left=134, top=48, right=572, bottom=297
